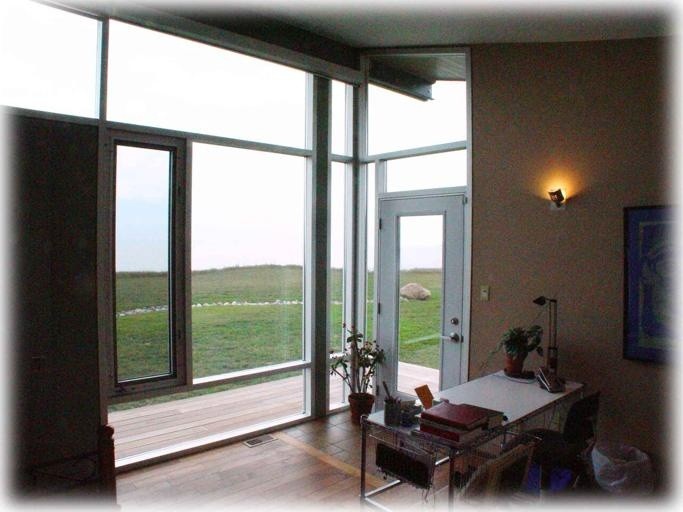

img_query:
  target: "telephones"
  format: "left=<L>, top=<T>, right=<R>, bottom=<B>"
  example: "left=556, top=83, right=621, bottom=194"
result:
left=535, top=366, right=565, bottom=393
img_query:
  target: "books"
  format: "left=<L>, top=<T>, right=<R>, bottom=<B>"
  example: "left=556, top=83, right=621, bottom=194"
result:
left=410, top=399, right=504, bottom=450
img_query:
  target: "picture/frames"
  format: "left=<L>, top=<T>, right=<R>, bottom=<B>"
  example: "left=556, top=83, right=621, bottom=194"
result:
left=622, top=204, right=683, bottom=365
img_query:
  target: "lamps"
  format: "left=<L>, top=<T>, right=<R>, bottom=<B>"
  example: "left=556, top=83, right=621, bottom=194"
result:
left=533, top=296, right=565, bottom=392
left=548, top=189, right=565, bottom=211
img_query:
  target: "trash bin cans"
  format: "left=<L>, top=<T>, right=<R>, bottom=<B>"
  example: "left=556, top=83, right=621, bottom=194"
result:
left=592, top=440, right=656, bottom=497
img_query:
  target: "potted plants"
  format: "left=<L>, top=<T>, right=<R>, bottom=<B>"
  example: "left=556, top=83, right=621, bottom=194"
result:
left=486, top=324, right=543, bottom=379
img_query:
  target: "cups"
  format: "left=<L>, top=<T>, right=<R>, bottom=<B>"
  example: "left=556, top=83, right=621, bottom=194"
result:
left=385, top=403, right=401, bottom=425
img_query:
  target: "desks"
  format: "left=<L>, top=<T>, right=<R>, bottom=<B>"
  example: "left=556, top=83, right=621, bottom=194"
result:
left=359, top=368, right=585, bottom=511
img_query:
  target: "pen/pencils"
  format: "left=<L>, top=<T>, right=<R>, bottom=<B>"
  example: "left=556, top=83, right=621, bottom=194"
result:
left=383, top=381, right=401, bottom=404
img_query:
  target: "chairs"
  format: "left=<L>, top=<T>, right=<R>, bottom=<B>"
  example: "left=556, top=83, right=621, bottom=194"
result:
left=455, top=441, right=536, bottom=502
left=500, top=390, right=602, bottom=490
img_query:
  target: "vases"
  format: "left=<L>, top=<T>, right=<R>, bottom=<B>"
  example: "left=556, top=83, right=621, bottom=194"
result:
left=348, top=393, right=376, bottom=420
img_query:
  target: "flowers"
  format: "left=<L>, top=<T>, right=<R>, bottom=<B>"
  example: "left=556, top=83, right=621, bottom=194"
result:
left=328, top=322, right=387, bottom=398
